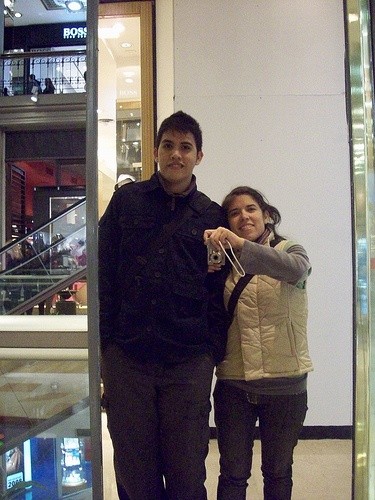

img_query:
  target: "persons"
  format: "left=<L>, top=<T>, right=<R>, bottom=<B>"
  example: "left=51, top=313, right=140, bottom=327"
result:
left=97, top=106, right=312, bottom=499
left=28, top=73, right=55, bottom=94
left=26, top=280, right=53, bottom=315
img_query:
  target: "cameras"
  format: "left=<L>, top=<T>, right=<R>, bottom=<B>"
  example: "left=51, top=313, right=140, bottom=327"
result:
left=207, top=238, right=225, bottom=265
